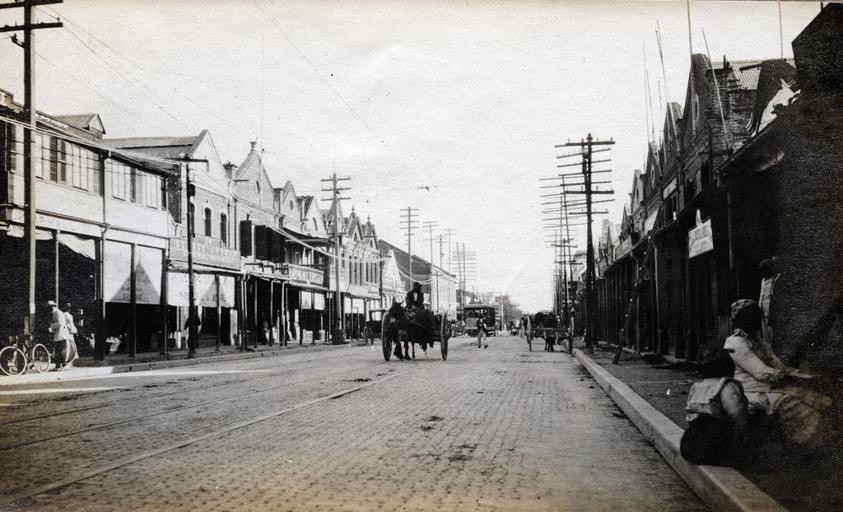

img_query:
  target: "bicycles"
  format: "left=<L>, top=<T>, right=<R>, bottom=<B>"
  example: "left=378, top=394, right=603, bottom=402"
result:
left=1, top=333, right=51, bottom=378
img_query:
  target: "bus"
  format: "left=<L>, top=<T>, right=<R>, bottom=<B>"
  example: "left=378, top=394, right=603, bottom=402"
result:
left=463, top=304, right=494, bottom=338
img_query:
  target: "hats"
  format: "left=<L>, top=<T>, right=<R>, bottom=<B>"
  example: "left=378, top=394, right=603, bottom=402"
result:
left=692, top=344, right=735, bottom=365
left=729, top=297, right=755, bottom=317
left=46, top=300, right=57, bottom=307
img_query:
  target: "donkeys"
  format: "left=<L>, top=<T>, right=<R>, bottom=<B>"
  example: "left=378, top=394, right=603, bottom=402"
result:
left=387, top=297, right=415, bottom=361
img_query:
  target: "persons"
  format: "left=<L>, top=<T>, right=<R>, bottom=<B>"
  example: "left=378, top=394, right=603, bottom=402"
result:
left=451, top=322, right=457, bottom=338
left=407, top=282, right=424, bottom=314
left=520, top=317, right=526, bottom=338
left=63, top=302, right=79, bottom=363
left=679, top=346, right=773, bottom=467
left=545, top=312, right=556, bottom=352
left=725, top=299, right=834, bottom=449
left=185, top=315, right=200, bottom=353
left=477, top=314, right=490, bottom=349
left=758, top=258, right=776, bottom=337
left=46, top=300, right=70, bottom=371
left=261, top=317, right=268, bottom=345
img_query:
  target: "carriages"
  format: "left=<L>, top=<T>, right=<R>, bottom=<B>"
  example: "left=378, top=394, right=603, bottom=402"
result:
left=378, top=301, right=453, bottom=363
left=525, top=312, right=575, bottom=355
